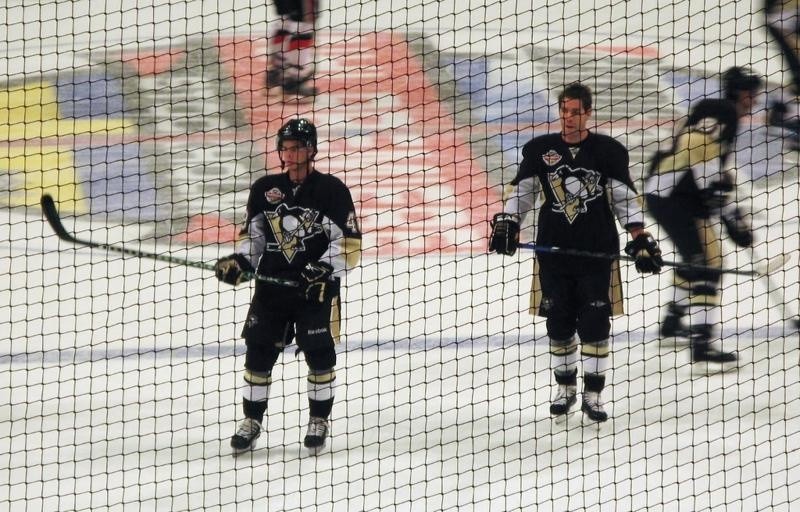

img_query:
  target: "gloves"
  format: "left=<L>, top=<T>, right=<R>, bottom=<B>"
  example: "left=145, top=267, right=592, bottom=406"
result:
left=625, top=233, right=662, bottom=274
left=298, top=261, right=334, bottom=304
left=214, top=253, right=255, bottom=285
left=721, top=209, right=753, bottom=247
left=488, top=212, right=521, bottom=256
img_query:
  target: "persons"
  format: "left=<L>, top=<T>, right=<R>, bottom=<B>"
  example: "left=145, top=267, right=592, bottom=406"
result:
left=763, top=0, right=799, bottom=151
left=642, top=66, right=762, bottom=364
left=489, top=82, right=662, bottom=424
left=215, top=118, right=362, bottom=451
left=265, top=0, right=320, bottom=95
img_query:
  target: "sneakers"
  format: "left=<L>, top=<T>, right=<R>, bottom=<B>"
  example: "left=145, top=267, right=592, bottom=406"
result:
left=694, top=346, right=735, bottom=361
left=265, top=69, right=282, bottom=89
left=285, top=76, right=320, bottom=96
left=663, top=324, right=692, bottom=337
left=550, top=384, right=577, bottom=415
left=581, top=390, right=607, bottom=422
left=304, top=416, right=331, bottom=447
left=231, top=417, right=264, bottom=449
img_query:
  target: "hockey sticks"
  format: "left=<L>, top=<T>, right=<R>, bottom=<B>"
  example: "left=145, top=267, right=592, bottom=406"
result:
left=518, top=244, right=790, bottom=276
left=746, top=246, right=800, bottom=331
left=40, top=195, right=308, bottom=288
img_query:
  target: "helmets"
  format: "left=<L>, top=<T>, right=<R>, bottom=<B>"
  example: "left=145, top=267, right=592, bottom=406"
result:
left=724, top=66, right=759, bottom=91
left=278, top=118, right=317, bottom=151
